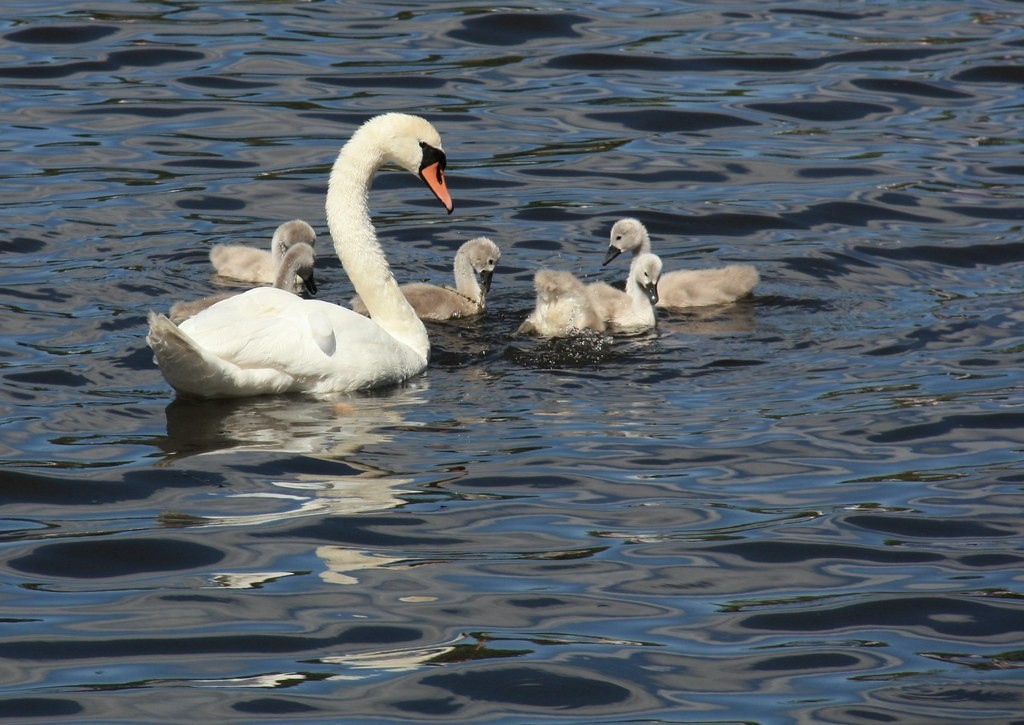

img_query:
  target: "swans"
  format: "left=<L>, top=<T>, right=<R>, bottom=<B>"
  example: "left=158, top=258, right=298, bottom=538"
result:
left=145, top=111, right=760, bottom=400
